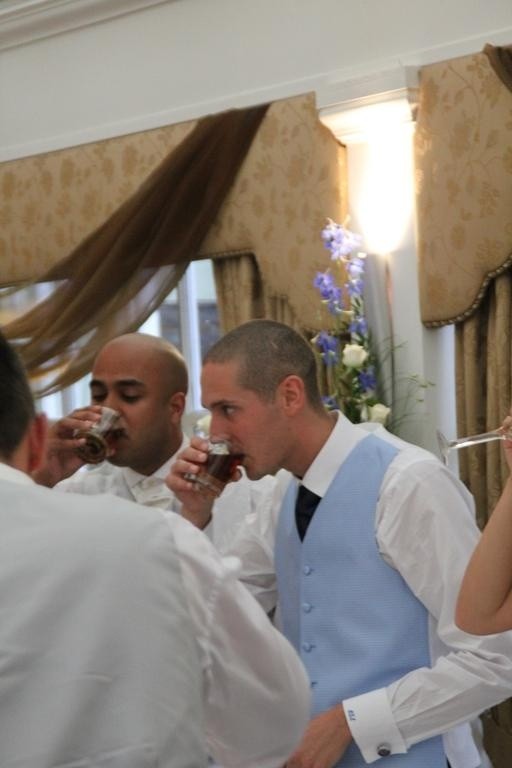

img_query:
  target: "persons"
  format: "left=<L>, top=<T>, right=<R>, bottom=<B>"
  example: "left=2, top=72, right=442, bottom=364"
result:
left=0, top=332, right=316, bottom=768
left=36, top=332, right=192, bottom=511
left=163, top=319, right=512, bottom=768
left=455, top=413, right=511, bottom=635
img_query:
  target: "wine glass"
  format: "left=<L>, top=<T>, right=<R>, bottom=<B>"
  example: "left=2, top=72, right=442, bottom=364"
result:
left=436, top=414, right=512, bottom=468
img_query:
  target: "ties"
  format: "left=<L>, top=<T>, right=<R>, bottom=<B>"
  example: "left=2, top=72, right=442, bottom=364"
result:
left=293, top=484, right=321, bottom=543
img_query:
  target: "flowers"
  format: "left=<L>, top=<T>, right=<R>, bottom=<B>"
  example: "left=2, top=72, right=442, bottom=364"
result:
left=300, top=214, right=438, bottom=437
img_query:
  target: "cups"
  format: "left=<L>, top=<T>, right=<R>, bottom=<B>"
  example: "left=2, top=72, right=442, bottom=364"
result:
left=177, top=429, right=240, bottom=500
left=70, top=404, right=127, bottom=464
